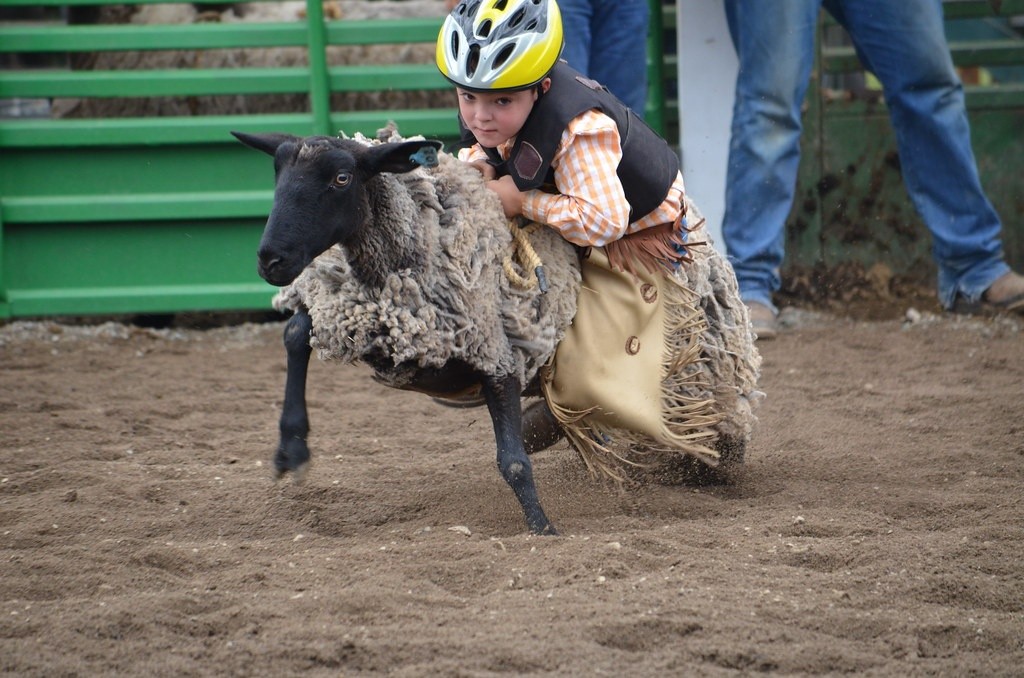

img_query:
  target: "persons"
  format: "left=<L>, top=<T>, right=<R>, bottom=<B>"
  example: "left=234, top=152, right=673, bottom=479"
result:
left=434, top=0, right=688, bottom=455
left=556, top=0, right=648, bottom=119
left=719, top=0, right=1024, bottom=338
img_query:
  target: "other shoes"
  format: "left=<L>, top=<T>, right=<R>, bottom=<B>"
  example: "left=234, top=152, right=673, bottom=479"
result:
left=956, top=270, right=1024, bottom=315
left=741, top=299, right=781, bottom=340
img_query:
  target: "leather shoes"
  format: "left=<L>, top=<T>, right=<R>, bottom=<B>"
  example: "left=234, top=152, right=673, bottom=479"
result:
left=433, top=392, right=487, bottom=407
left=520, top=397, right=567, bottom=455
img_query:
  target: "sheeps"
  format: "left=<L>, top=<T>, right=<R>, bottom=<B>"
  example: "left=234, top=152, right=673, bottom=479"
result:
left=230, top=128, right=763, bottom=540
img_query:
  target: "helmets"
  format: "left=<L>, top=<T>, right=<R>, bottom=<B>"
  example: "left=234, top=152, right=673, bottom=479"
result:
left=434, top=0, right=567, bottom=93
